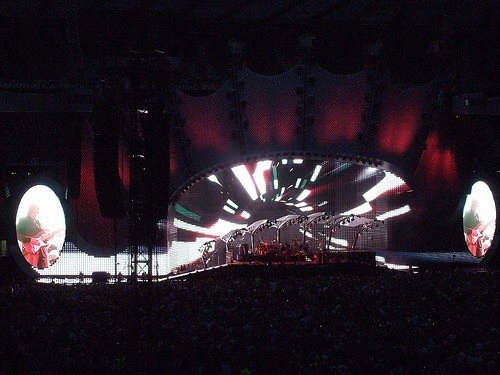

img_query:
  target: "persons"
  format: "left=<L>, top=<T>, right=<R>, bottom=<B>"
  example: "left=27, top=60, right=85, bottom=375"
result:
left=463, top=200, right=494, bottom=256
left=17, top=203, right=54, bottom=268
left=0, top=240, right=500, bottom=375
